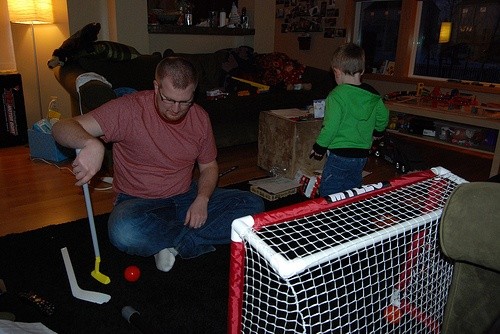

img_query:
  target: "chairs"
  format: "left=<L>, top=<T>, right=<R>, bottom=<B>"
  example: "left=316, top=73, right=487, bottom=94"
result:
left=440, top=181, right=500, bottom=334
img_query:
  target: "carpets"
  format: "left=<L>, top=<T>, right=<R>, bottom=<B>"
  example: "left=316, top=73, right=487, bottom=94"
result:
left=0, top=173, right=500, bottom=334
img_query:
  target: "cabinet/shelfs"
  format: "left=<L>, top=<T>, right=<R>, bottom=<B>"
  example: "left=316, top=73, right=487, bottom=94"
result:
left=384, top=94, right=500, bottom=178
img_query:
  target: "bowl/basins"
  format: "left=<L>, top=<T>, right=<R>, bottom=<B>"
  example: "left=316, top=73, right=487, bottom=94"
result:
left=157, top=15, right=180, bottom=26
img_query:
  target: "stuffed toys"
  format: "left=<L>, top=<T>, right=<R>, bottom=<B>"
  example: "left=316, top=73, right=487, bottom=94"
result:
left=276, top=62, right=312, bottom=91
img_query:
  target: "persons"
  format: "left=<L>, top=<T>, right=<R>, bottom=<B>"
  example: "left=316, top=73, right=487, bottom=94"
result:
left=310, top=43, right=389, bottom=197
left=52, top=57, right=265, bottom=260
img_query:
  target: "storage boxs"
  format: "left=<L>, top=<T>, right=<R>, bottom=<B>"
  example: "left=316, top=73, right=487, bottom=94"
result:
left=257, top=111, right=326, bottom=180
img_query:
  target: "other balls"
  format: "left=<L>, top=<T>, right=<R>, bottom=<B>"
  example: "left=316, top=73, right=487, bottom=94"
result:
left=124, top=267, right=140, bottom=282
left=385, top=302, right=401, bottom=322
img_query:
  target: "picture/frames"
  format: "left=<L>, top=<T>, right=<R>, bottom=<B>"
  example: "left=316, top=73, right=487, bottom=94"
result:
left=275, top=0, right=347, bottom=39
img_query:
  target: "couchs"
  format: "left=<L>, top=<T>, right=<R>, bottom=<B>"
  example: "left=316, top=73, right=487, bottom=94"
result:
left=52, top=49, right=328, bottom=173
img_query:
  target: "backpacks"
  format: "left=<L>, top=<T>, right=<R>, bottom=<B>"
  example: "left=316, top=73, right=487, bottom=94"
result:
left=222, top=46, right=261, bottom=92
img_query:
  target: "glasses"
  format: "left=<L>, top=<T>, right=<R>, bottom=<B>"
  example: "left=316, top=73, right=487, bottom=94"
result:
left=158, top=83, right=195, bottom=106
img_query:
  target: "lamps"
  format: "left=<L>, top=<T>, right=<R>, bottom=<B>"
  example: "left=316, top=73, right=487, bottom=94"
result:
left=436, top=17, right=455, bottom=48
left=8, top=0, right=55, bottom=120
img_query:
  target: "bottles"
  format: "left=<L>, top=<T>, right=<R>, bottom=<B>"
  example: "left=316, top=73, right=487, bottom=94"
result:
left=184, top=2, right=193, bottom=26
left=240, top=7, right=248, bottom=28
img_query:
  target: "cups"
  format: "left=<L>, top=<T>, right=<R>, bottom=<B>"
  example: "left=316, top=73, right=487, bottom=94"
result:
left=210, top=11, right=217, bottom=28
